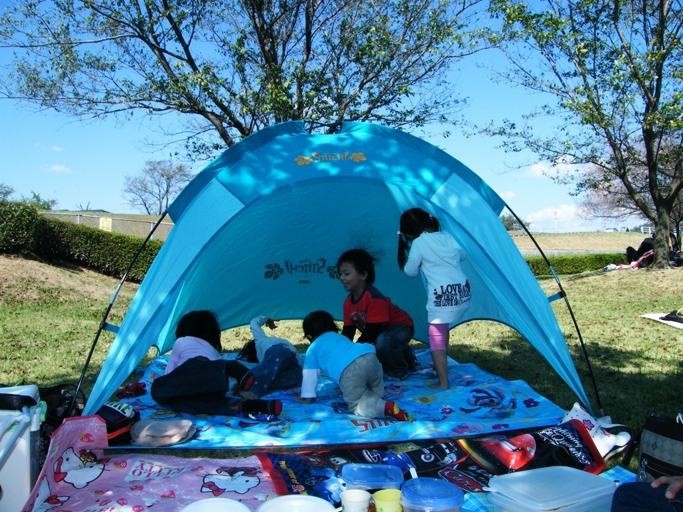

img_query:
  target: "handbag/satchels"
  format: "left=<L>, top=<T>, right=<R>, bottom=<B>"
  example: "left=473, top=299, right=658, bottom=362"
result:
left=131, top=420, right=196, bottom=447
left=95, top=400, right=140, bottom=442
left=638, top=416, right=683, bottom=479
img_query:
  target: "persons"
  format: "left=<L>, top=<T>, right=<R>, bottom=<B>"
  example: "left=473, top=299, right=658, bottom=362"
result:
left=296, top=310, right=411, bottom=422
left=395, top=208, right=473, bottom=390
left=334, top=245, right=416, bottom=381
left=236, top=314, right=304, bottom=403
left=625, top=232, right=656, bottom=267
left=611, top=474, right=682, bottom=511
left=150, top=309, right=282, bottom=417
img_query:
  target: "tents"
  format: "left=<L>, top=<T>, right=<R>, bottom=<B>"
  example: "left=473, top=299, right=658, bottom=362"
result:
left=58, top=117, right=610, bottom=461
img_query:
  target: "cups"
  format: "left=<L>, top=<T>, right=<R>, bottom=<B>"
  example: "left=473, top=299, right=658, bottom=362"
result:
left=339, top=488, right=372, bottom=512
left=371, top=488, right=403, bottom=512
